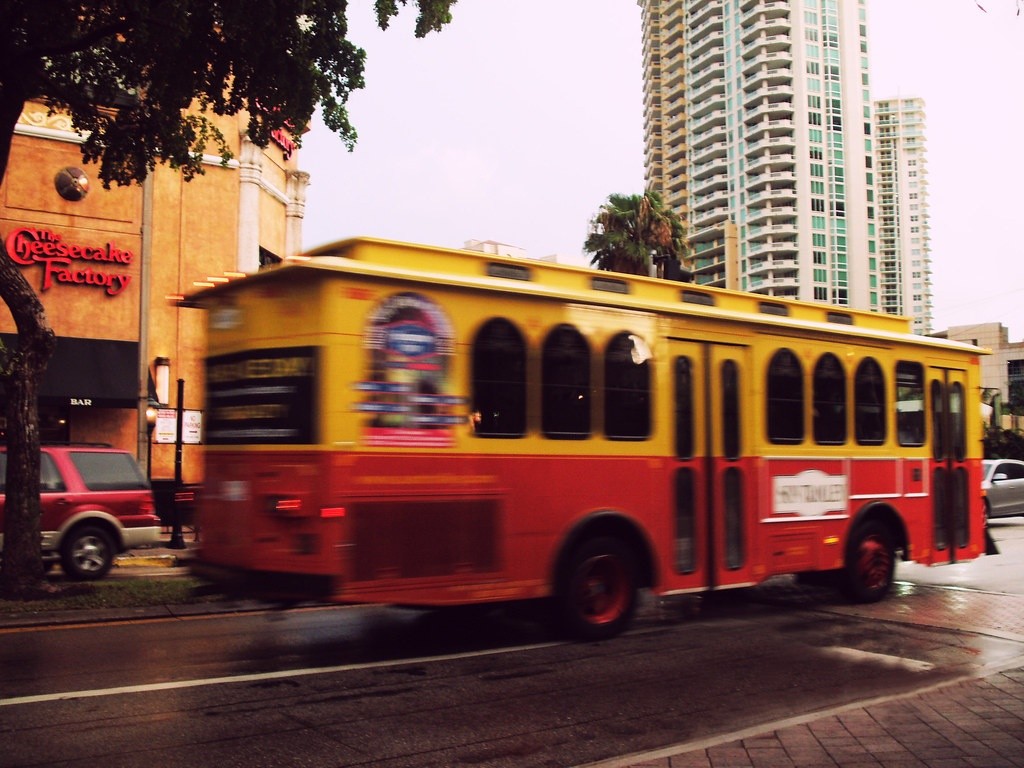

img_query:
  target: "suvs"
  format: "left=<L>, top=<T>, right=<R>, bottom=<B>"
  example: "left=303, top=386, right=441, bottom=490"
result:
left=0, top=441, right=165, bottom=583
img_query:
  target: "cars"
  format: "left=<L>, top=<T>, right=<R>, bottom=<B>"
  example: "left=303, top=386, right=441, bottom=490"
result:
left=980, top=459, right=1024, bottom=522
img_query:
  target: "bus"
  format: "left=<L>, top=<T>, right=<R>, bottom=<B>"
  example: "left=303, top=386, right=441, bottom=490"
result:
left=167, top=235, right=1003, bottom=638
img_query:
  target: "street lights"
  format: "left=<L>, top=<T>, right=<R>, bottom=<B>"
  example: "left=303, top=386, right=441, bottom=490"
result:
left=145, top=399, right=164, bottom=483
